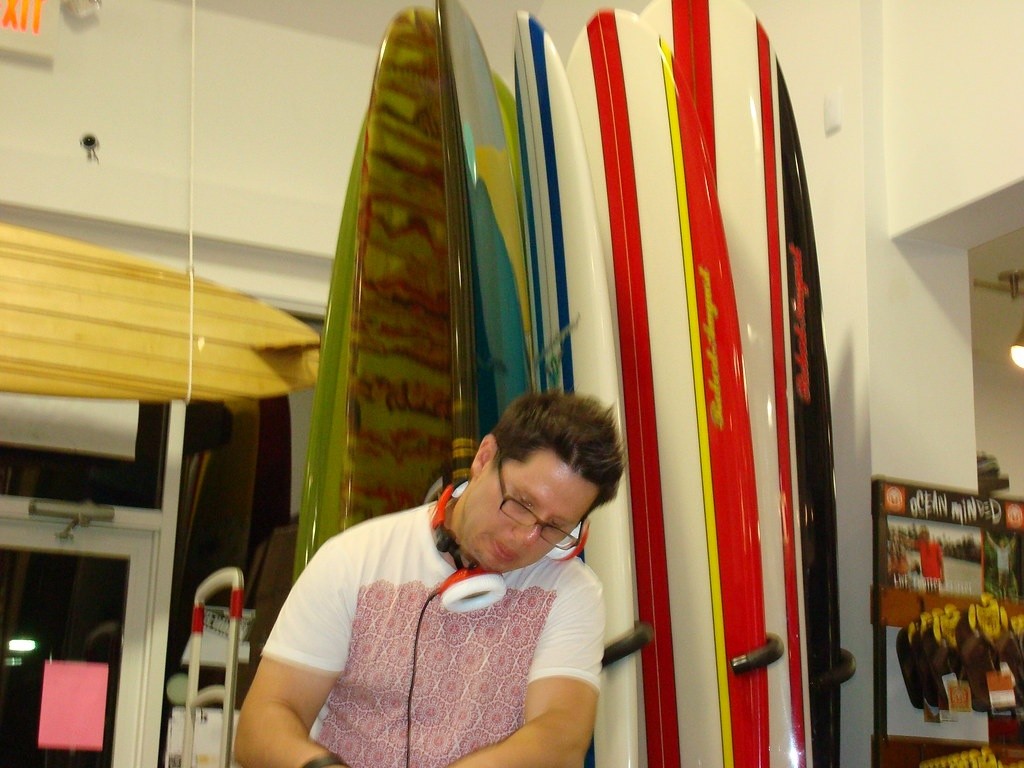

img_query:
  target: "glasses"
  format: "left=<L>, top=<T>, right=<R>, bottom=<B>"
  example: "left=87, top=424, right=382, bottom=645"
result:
left=495, top=450, right=586, bottom=550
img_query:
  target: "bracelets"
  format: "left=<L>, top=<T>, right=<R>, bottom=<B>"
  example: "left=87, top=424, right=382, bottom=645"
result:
left=302, top=752, right=350, bottom=768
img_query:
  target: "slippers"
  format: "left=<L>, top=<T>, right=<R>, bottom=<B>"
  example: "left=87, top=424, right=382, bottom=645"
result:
left=895, top=611, right=1024, bottom=713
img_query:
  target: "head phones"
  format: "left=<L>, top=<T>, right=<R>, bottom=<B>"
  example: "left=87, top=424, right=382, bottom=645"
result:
left=432, top=476, right=592, bottom=613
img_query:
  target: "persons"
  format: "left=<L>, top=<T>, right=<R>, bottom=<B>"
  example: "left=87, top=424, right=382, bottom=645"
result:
left=233, top=390, right=626, bottom=768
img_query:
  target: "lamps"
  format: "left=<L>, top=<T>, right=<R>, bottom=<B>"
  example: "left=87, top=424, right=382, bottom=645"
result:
left=0, top=0, right=100, bottom=65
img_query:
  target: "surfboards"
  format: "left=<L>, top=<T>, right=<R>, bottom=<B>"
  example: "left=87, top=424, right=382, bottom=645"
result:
left=1, top=222, right=322, bottom=402
left=291, top=0, right=840, bottom=768
